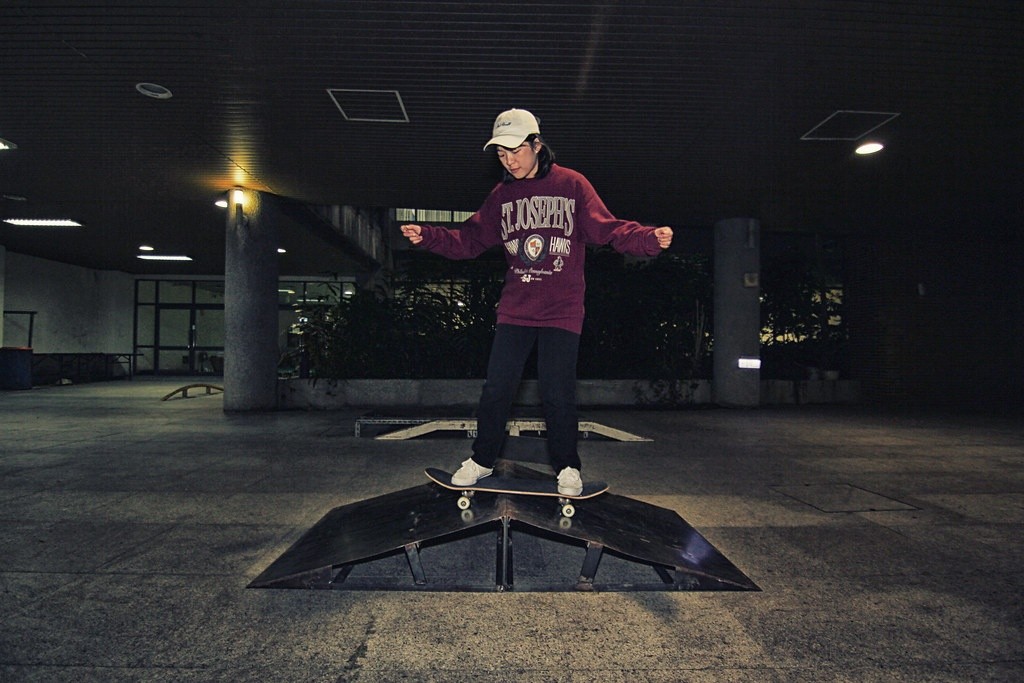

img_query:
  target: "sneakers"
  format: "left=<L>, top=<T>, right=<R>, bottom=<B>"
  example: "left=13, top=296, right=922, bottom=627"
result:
left=451, top=458, right=494, bottom=486
left=557, top=466, right=583, bottom=496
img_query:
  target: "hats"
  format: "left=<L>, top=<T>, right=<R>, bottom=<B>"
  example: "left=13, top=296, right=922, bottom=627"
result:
left=482, top=108, right=541, bottom=152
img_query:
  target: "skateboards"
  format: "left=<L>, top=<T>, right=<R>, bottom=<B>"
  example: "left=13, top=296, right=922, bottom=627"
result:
left=424, top=468, right=608, bottom=516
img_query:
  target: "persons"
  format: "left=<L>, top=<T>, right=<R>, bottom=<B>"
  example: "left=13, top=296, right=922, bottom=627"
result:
left=400, top=106, right=673, bottom=496
